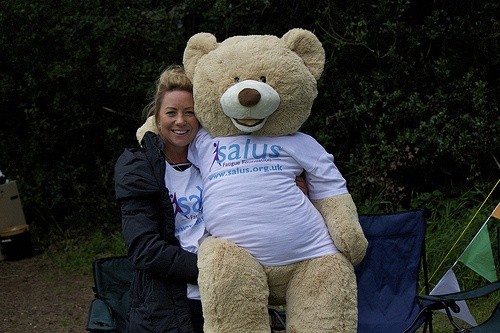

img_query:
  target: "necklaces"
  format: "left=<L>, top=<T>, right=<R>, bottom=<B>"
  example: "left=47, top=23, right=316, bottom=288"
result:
left=163, top=151, right=191, bottom=172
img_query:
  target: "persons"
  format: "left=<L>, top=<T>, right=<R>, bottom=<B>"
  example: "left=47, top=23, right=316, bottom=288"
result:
left=114, top=64, right=308, bottom=333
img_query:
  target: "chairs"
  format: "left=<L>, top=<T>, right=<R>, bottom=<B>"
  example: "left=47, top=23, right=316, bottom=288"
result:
left=354, top=208, right=432, bottom=333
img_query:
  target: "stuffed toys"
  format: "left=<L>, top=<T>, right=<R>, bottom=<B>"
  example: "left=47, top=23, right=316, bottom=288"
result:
left=182, top=28, right=369, bottom=333
